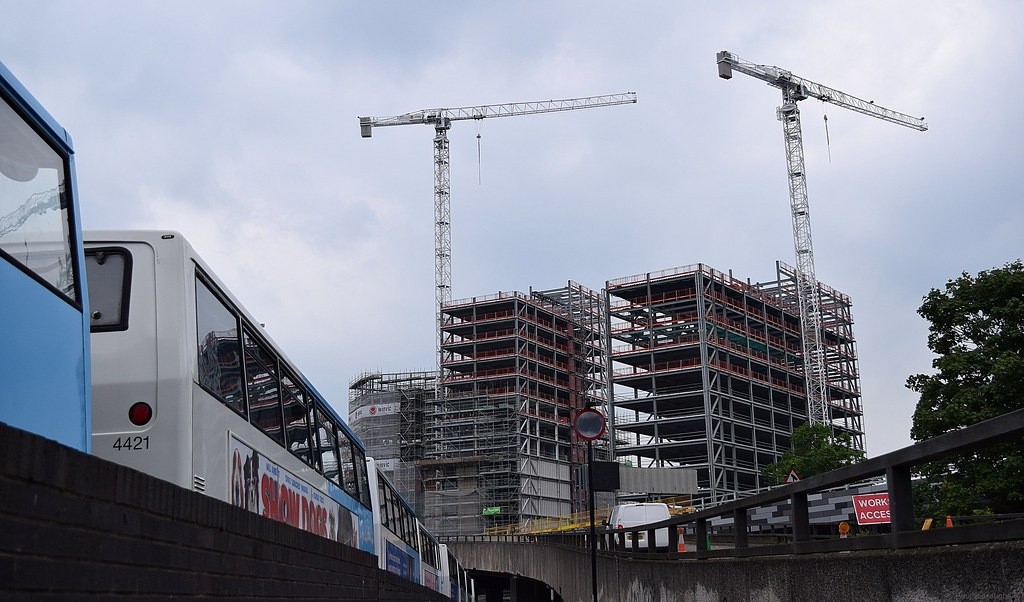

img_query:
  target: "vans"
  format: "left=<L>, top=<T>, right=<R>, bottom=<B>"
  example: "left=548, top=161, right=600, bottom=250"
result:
left=602, top=502, right=671, bottom=547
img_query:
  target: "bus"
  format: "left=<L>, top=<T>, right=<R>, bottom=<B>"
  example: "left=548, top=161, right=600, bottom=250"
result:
left=1, top=64, right=477, bottom=602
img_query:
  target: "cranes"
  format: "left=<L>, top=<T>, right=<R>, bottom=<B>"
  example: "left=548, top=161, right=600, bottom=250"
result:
left=359, top=90, right=637, bottom=381
left=717, top=50, right=929, bottom=441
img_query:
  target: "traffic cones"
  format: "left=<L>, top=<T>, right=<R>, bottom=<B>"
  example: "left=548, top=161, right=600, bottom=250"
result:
left=946, top=516, right=953, bottom=527
left=678, top=533, right=686, bottom=553
